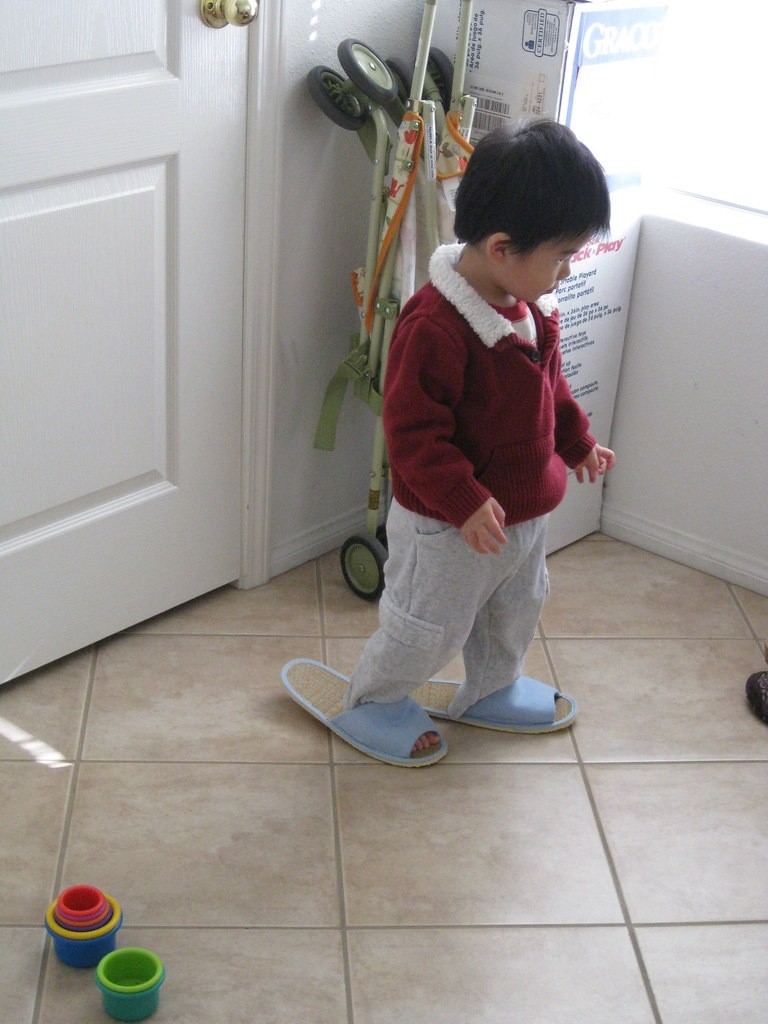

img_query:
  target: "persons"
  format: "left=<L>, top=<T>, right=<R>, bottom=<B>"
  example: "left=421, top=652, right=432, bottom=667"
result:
left=279, top=117, right=617, bottom=768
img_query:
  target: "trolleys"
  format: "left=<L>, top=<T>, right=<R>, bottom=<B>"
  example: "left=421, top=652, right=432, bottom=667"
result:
left=306, top=1, right=479, bottom=599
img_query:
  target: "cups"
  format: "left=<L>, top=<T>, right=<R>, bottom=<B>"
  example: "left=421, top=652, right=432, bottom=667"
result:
left=94, top=946, right=165, bottom=1022
left=44, top=884, right=122, bottom=969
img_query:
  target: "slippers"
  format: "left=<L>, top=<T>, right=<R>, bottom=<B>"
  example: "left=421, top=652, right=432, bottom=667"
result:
left=407, top=676, right=579, bottom=733
left=281, top=657, right=448, bottom=767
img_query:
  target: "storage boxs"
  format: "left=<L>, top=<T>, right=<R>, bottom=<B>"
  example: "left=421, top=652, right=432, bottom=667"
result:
left=397, top=1, right=661, bottom=564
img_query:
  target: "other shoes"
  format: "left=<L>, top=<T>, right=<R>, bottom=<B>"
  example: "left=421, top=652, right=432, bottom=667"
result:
left=745, top=671, right=768, bottom=725
